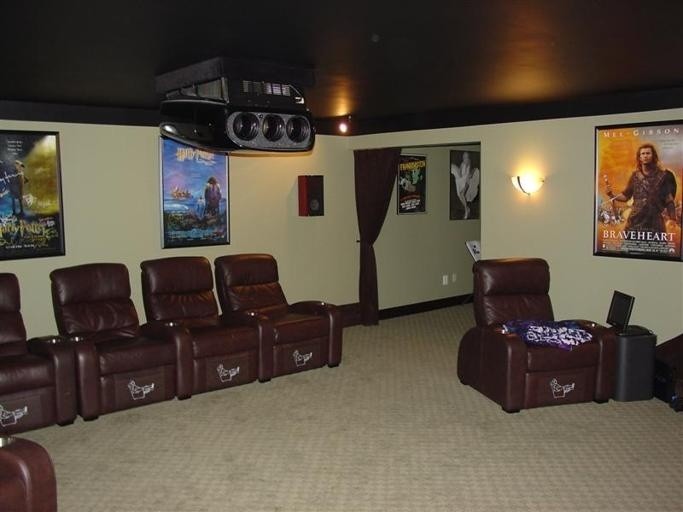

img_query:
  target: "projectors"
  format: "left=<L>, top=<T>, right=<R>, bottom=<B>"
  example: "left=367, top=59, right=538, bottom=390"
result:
left=154, top=96, right=316, bottom=158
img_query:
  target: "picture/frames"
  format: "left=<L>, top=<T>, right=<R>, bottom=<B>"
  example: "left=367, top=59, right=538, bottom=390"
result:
left=160, top=134, right=232, bottom=251
left=397, top=152, right=429, bottom=216
left=1, top=128, right=68, bottom=261
left=591, top=119, right=683, bottom=262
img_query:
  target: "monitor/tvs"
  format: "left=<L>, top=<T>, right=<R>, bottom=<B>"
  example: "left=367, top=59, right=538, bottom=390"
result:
left=606, top=289, right=635, bottom=332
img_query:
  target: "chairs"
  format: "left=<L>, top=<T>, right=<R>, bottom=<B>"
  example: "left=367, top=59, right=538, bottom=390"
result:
left=455, top=256, right=612, bottom=417
left=1, top=433, right=57, bottom=512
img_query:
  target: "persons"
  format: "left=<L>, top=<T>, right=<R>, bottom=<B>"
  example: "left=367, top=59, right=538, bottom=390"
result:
left=399, top=158, right=423, bottom=193
left=203, top=176, right=221, bottom=216
left=450, top=150, right=480, bottom=219
left=604, top=142, right=677, bottom=254
left=195, top=196, right=205, bottom=217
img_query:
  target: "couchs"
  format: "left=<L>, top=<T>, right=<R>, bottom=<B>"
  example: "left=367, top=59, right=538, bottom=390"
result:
left=48, top=261, right=176, bottom=424
left=213, top=252, right=345, bottom=385
left=138, top=254, right=276, bottom=400
left=1, top=269, right=79, bottom=437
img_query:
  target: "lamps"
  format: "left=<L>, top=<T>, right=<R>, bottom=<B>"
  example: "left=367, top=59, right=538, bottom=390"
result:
left=510, top=172, right=546, bottom=196
